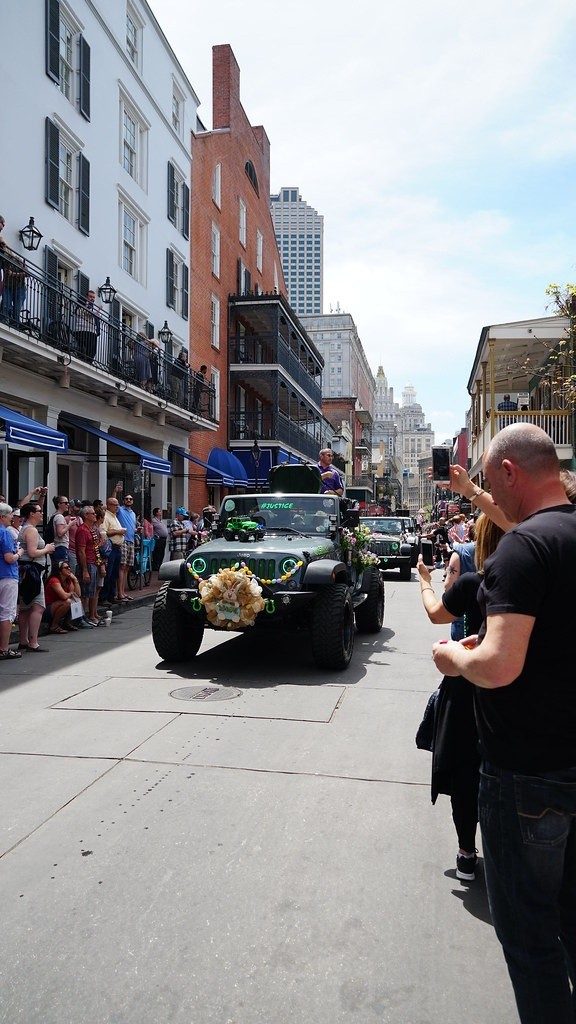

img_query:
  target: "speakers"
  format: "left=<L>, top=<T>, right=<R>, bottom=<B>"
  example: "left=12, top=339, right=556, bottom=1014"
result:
left=269, top=463, right=322, bottom=494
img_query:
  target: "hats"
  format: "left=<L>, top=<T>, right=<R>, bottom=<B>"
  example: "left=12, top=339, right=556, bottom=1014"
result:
left=69, top=498, right=84, bottom=507
left=292, top=514, right=304, bottom=521
left=12, top=507, right=21, bottom=517
left=313, top=511, right=330, bottom=520
left=81, top=499, right=93, bottom=506
left=176, top=507, right=189, bottom=516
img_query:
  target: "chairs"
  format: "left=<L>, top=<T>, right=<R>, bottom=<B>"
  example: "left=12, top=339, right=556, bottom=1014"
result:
left=9, top=304, right=43, bottom=337
left=49, top=321, right=86, bottom=361
left=111, top=353, right=137, bottom=383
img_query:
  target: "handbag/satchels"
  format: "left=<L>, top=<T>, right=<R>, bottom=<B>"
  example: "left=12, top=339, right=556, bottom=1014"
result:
left=43, top=514, right=58, bottom=545
left=70, top=593, right=84, bottom=620
left=99, top=532, right=113, bottom=558
left=17, top=558, right=46, bottom=596
left=416, top=687, right=440, bottom=751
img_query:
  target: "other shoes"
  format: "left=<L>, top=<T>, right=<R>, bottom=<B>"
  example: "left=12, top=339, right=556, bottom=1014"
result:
left=0, top=594, right=134, bottom=660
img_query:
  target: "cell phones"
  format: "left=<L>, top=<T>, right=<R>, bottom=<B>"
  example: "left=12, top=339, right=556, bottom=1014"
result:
left=40, top=488, right=46, bottom=493
left=420, top=540, right=434, bottom=570
left=118, top=481, right=123, bottom=489
left=432, top=445, right=450, bottom=484
left=439, top=540, right=446, bottom=544
left=137, top=527, right=142, bottom=533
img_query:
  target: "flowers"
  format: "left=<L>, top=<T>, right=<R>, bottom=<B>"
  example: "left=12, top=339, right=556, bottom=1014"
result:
left=338, top=522, right=382, bottom=572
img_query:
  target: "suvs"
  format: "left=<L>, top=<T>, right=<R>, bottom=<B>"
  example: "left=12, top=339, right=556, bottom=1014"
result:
left=356, top=515, right=422, bottom=582
left=152, top=464, right=386, bottom=673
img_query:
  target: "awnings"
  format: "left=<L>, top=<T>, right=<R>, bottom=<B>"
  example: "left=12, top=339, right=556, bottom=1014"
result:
left=0, top=405, right=68, bottom=453
left=206, top=448, right=248, bottom=488
left=169, top=447, right=234, bottom=487
left=59, top=415, right=172, bottom=476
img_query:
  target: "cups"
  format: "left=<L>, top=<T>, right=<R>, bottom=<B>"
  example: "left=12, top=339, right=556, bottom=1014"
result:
left=137, top=527, right=142, bottom=533
left=71, top=517, right=75, bottom=526
left=106, top=611, right=112, bottom=618
left=106, top=618, right=111, bottom=623
left=105, top=619, right=110, bottom=626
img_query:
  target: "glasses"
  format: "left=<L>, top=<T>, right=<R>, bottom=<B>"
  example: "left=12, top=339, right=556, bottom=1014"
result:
left=32, top=509, right=42, bottom=514
left=125, top=498, right=133, bottom=501
left=62, top=566, right=70, bottom=570
left=57, top=501, right=69, bottom=505
left=87, top=512, right=96, bottom=515
left=322, top=453, right=334, bottom=458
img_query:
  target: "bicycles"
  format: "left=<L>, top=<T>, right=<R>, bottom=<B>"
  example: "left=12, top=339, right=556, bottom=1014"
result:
left=126, top=534, right=159, bottom=591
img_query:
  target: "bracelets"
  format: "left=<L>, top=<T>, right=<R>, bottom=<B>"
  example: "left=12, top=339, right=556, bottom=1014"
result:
left=468, top=490, right=484, bottom=501
left=421, top=588, right=434, bottom=594
left=17, top=553, right=21, bottom=558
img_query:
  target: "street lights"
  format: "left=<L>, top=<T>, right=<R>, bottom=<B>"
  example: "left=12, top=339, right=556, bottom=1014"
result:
left=251, top=437, right=262, bottom=493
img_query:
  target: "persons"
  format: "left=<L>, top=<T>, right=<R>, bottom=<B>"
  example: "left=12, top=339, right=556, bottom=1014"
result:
left=416, top=424, right=576, bottom=1024
left=257, top=510, right=327, bottom=532
left=170, top=352, right=190, bottom=407
left=191, top=366, right=209, bottom=415
left=74, top=291, right=102, bottom=362
left=0, top=216, right=27, bottom=328
left=473, top=394, right=531, bottom=440
left=135, top=332, right=160, bottom=392
left=0, top=483, right=217, bottom=661
left=316, top=449, right=344, bottom=495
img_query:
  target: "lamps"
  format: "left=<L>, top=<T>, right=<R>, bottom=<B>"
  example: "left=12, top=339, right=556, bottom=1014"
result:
left=59, top=374, right=71, bottom=389
left=106, top=395, right=117, bottom=407
left=158, top=320, right=172, bottom=343
left=158, top=412, right=166, bottom=426
left=134, top=402, right=142, bottom=416
left=19, top=217, right=44, bottom=250
left=97, top=277, right=117, bottom=304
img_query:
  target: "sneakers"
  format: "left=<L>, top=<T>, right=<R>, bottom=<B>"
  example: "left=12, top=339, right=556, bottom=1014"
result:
left=456, top=849, right=478, bottom=881
